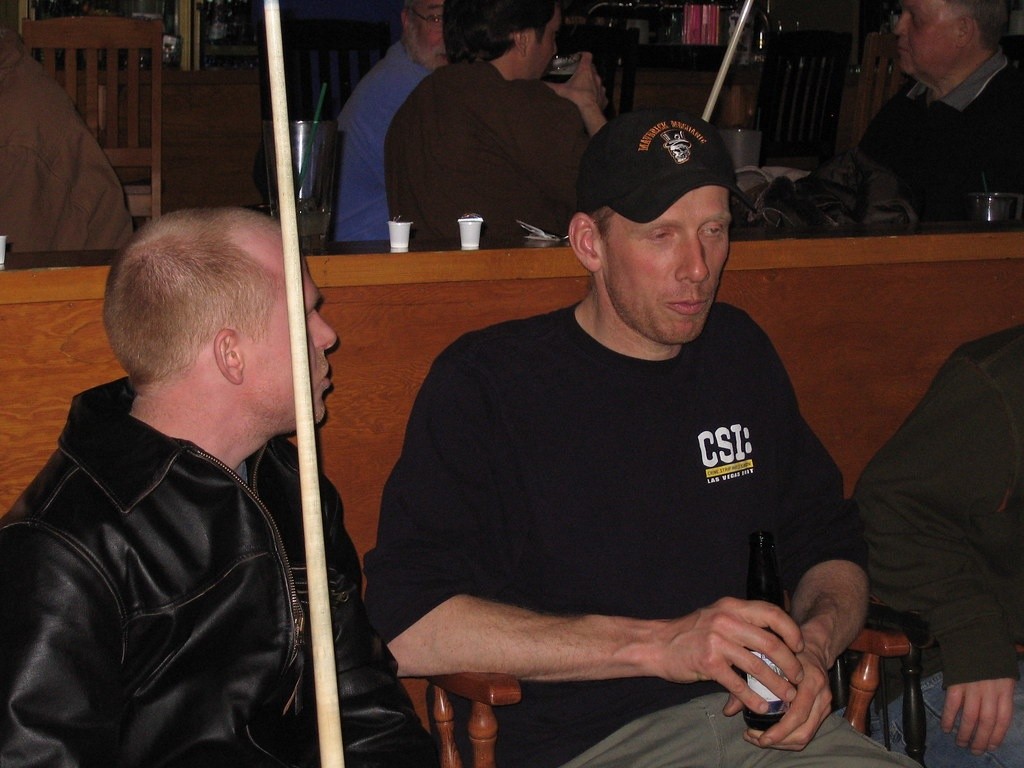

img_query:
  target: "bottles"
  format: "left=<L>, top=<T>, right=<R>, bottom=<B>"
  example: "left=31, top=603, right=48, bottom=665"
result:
left=742, top=531, right=797, bottom=730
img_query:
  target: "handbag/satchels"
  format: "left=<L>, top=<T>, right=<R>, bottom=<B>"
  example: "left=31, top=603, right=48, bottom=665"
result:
left=730, top=156, right=918, bottom=242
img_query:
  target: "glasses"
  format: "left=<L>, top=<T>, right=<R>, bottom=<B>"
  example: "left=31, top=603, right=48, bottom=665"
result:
left=409, top=8, right=445, bottom=30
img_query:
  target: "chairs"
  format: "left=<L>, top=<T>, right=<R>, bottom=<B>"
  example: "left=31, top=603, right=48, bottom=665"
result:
left=850, top=32, right=908, bottom=152
left=758, top=28, right=853, bottom=166
left=22, top=16, right=163, bottom=223
left=428, top=595, right=913, bottom=768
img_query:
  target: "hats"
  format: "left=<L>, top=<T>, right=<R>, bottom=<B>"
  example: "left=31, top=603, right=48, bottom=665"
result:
left=576, top=106, right=757, bottom=223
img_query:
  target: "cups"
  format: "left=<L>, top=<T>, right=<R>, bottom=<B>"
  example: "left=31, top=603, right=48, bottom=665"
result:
left=458, top=218, right=483, bottom=250
left=388, top=221, right=413, bottom=253
left=542, top=54, right=581, bottom=83
left=626, top=19, right=649, bottom=44
left=262, top=118, right=340, bottom=254
left=0, top=235, right=7, bottom=271
left=967, top=192, right=1024, bottom=221
left=717, top=129, right=764, bottom=170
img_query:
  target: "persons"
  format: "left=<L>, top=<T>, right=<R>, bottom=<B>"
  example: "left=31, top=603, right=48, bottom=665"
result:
left=330, top=0, right=451, bottom=239
left=360, top=109, right=926, bottom=768
left=853, top=0, right=1023, bottom=225
left=0, top=207, right=441, bottom=767
left=382, top=0, right=609, bottom=251
left=852, top=323, right=1024, bottom=767
left=0, top=27, right=133, bottom=253
